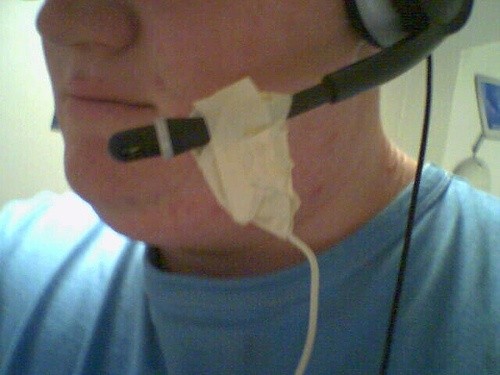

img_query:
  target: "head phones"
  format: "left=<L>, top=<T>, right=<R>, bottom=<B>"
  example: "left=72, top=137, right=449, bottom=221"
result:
left=346, top=0, right=474, bottom=54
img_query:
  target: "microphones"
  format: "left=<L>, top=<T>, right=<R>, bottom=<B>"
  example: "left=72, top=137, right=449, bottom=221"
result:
left=110, top=22, right=448, bottom=160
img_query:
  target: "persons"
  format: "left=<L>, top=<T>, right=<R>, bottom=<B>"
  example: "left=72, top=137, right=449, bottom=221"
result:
left=0, top=0, right=499, bottom=375
